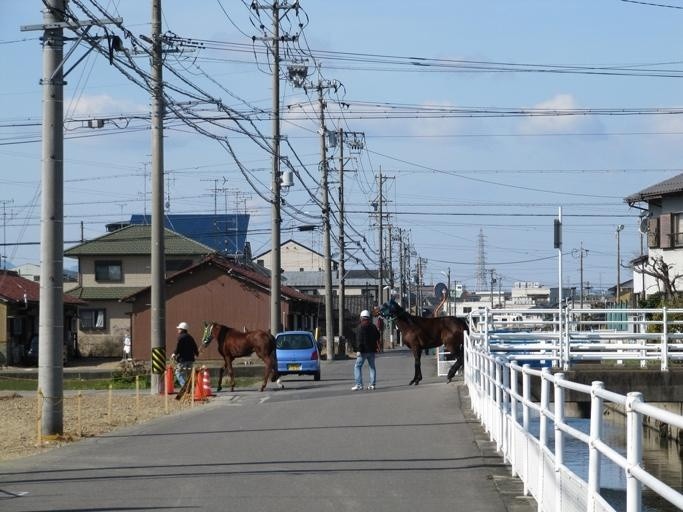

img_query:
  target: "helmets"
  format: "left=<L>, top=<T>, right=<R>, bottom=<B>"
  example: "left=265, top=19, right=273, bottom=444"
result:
left=360, top=309, right=371, bottom=318
left=176, top=322, right=189, bottom=330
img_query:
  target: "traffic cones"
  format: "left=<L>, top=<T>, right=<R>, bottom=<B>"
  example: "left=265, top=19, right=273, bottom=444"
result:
left=160, top=364, right=179, bottom=395
left=193, top=368, right=217, bottom=401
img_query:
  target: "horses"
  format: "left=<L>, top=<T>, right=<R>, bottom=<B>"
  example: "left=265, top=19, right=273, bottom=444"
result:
left=201, top=320, right=278, bottom=392
left=380, top=296, right=469, bottom=386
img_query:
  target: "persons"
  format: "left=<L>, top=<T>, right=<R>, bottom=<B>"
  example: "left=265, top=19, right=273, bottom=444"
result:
left=168, top=321, right=199, bottom=395
left=350, top=309, right=381, bottom=391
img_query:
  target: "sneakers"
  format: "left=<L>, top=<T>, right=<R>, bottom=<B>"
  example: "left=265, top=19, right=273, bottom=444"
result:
left=351, top=384, right=363, bottom=390
left=367, top=386, right=375, bottom=390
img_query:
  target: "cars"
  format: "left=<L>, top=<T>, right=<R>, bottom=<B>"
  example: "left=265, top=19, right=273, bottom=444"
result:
left=271, top=331, right=320, bottom=382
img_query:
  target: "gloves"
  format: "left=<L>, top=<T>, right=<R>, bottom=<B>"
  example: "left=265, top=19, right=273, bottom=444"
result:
left=356, top=351, right=361, bottom=357
